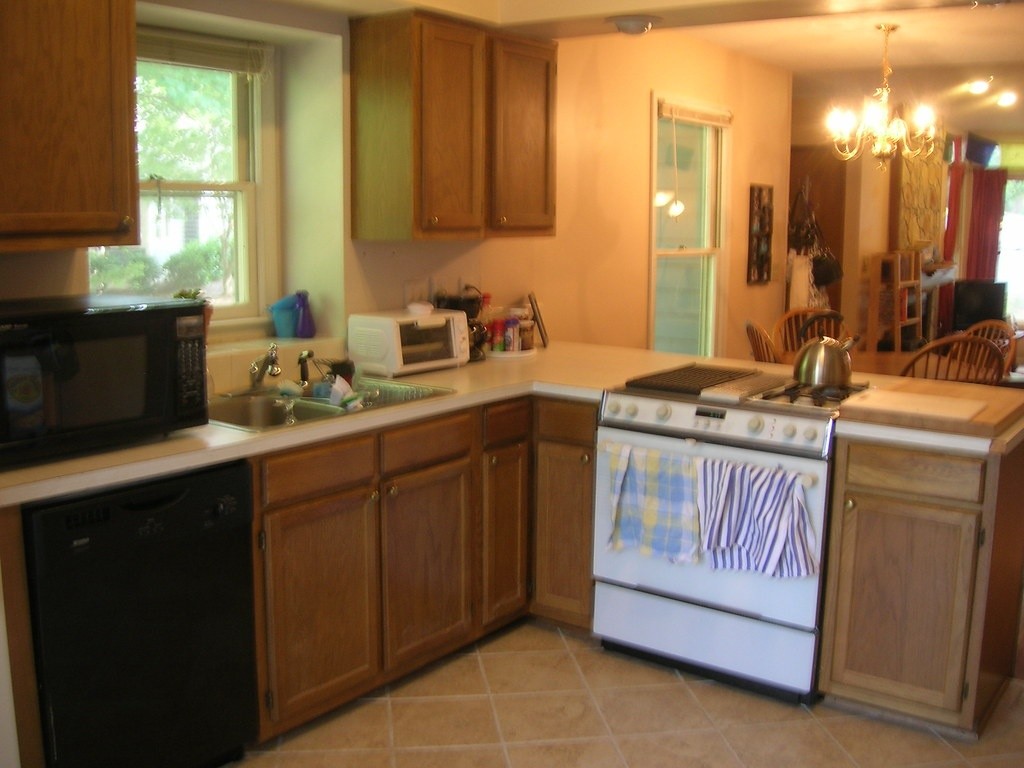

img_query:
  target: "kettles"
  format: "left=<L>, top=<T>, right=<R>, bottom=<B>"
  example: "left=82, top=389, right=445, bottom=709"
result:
left=793, top=312, right=862, bottom=387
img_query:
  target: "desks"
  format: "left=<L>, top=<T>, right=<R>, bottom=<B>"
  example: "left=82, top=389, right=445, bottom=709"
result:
left=780, top=352, right=1024, bottom=389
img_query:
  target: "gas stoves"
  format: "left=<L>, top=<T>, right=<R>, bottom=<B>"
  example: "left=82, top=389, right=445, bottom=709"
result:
left=603, top=363, right=870, bottom=458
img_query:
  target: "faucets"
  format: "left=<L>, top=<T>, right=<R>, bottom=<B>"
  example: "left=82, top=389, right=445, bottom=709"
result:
left=247, top=342, right=282, bottom=388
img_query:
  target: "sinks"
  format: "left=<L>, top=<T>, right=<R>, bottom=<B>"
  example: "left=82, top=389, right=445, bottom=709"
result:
left=198, top=393, right=345, bottom=434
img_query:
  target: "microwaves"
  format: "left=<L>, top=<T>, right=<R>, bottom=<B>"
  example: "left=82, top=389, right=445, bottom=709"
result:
left=0, top=294, right=210, bottom=472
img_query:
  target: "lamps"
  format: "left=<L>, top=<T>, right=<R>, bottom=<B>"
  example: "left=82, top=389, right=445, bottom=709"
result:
left=824, top=22, right=936, bottom=171
left=605, top=14, right=664, bottom=35
left=966, top=131, right=1002, bottom=169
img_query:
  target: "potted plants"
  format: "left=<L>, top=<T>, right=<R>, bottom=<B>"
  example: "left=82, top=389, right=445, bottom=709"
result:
left=171, top=288, right=213, bottom=347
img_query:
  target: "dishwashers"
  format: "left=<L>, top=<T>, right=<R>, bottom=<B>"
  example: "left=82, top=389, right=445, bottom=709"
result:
left=21, top=456, right=261, bottom=767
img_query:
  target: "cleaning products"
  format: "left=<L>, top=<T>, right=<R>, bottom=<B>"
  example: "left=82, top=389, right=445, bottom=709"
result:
left=294, top=288, right=316, bottom=338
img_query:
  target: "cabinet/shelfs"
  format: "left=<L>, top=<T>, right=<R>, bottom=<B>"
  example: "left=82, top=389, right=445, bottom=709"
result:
left=867, top=250, right=922, bottom=353
left=251, top=409, right=480, bottom=747
left=530, top=396, right=599, bottom=629
left=476, top=395, right=534, bottom=641
left=485, top=30, right=560, bottom=240
left=0, top=0, right=141, bottom=253
left=349, top=7, right=485, bottom=241
left=817, top=436, right=1024, bottom=743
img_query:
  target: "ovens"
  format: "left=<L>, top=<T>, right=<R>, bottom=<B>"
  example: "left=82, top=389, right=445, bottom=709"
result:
left=348, top=307, right=470, bottom=378
left=592, top=426, right=830, bottom=703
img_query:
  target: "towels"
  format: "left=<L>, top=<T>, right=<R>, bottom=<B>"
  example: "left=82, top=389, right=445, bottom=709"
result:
left=602, top=443, right=817, bottom=577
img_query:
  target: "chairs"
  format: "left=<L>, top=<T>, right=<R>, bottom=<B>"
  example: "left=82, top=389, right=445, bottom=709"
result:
left=898, top=334, right=1005, bottom=386
left=745, top=320, right=783, bottom=366
left=945, top=320, right=1018, bottom=375
left=773, top=306, right=857, bottom=353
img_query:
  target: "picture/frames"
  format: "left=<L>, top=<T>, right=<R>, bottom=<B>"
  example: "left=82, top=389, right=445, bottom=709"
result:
left=528, top=291, right=550, bottom=348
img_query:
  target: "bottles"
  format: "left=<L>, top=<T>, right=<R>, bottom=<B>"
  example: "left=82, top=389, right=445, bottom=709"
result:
left=478, top=292, right=521, bottom=351
